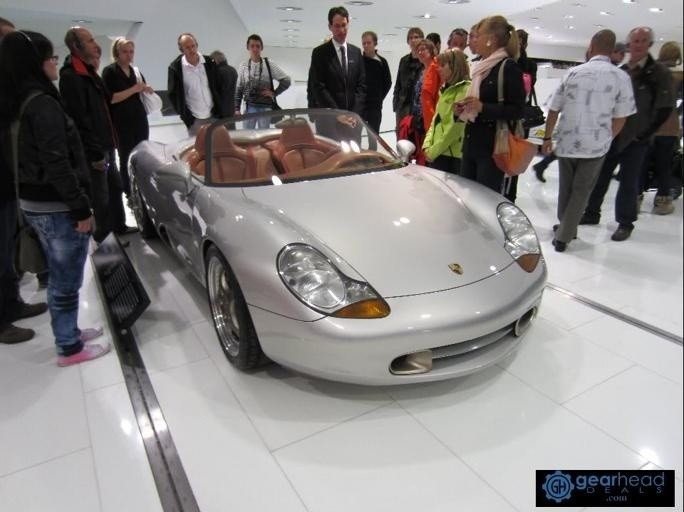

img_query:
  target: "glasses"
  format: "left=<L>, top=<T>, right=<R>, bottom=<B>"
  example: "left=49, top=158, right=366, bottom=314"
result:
left=182, top=45, right=196, bottom=50
left=45, top=54, right=58, bottom=62
left=474, top=32, right=489, bottom=38
left=416, top=48, right=429, bottom=53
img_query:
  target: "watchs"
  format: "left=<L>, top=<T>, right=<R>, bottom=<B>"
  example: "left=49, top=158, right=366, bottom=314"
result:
left=544, top=137, right=552, bottom=141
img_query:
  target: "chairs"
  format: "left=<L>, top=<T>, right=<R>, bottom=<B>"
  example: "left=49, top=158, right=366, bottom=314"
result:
left=187, top=124, right=257, bottom=182
left=266, top=118, right=342, bottom=174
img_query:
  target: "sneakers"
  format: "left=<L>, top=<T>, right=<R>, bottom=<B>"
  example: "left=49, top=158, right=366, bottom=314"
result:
left=57, top=344, right=110, bottom=367
left=578, top=214, right=600, bottom=225
left=10, top=303, right=47, bottom=323
left=652, top=192, right=674, bottom=215
left=611, top=224, right=634, bottom=241
left=120, top=240, right=129, bottom=247
left=119, top=226, right=139, bottom=234
left=635, top=195, right=644, bottom=214
left=37, top=269, right=50, bottom=288
left=1, top=324, right=35, bottom=343
left=79, top=326, right=103, bottom=343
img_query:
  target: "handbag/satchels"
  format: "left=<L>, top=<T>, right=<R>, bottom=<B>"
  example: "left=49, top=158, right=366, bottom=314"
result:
left=522, top=83, right=545, bottom=128
left=134, top=67, right=163, bottom=115
left=12, top=92, right=51, bottom=274
left=492, top=58, right=538, bottom=176
left=265, top=57, right=282, bottom=111
left=438, top=117, right=462, bottom=172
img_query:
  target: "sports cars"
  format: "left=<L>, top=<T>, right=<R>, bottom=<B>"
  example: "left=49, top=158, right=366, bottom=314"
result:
left=127, top=108, right=549, bottom=388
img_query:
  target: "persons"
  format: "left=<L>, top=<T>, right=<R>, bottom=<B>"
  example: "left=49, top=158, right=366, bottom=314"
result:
left=0, top=17, right=49, bottom=345
left=428, top=33, right=441, bottom=55
left=469, top=25, right=482, bottom=62
left=0, top=29, right=112, bottom=368
left=580, top=27, right=677, bottom=241
left=102, top=37, right=154, bottom=199
left=453, top=15, right=527, bottom=198
left=504, top=28, right=537, bottom=201
left=59, top=27, right=139, bottom=247
left=167, top=33, right=224, bottom=138
left=542, top=29, right=637, bottom=252
left=409, top=39, right=445, bottom=165
left=307, top=36, right=333, bottom=135
left=394, top=28, right=424, bottom=142
left=533, top=39, right=625, bottom=183
left=312, top=7, right=368, bottom=151
left=422, top=47, right=471, bottom=175
left=361, top=31, right=392, bottom=150
left=634, top=40, right=684, bottom=215
left=89, top=45, right=102, bottom=72
left=234, top=34, right=291, bottom=129
left=211, top=51, right=238, bottom=131
left=448, top=28, right=468, bottom=51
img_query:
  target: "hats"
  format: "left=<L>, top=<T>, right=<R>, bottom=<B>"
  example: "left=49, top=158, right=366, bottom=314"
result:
left=613, top=43, right=630, bottom=52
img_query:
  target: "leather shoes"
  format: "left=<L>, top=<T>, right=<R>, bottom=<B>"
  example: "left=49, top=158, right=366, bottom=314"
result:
left=552, top=239, right=566, bottom=251
left=553, top=225, right=577, bottom=239
left=533, top=161, right=547, bottom=182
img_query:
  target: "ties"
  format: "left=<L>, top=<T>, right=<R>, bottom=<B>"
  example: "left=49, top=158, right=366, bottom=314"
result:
left=339, top=46, right=346, bottom=77
